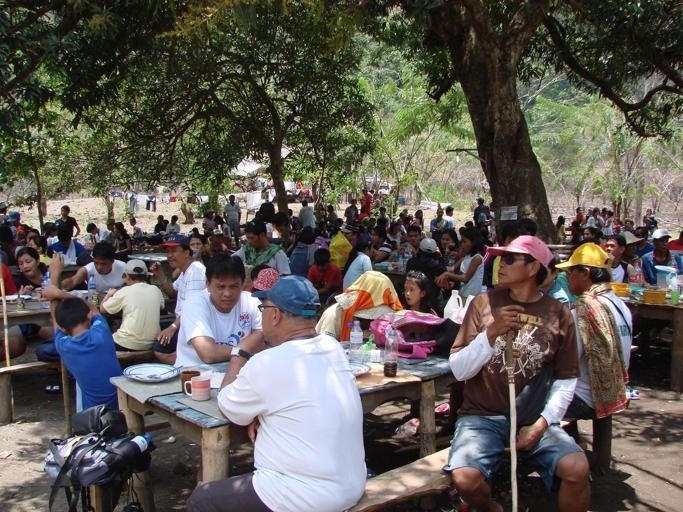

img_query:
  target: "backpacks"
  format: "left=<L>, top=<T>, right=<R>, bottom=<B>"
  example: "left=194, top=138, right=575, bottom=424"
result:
left=370, top=309, right=444, bottom=358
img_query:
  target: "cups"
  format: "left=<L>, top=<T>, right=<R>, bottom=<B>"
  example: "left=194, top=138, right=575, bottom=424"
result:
left=183, top=376, right=210, bottom=401
left=370, top=349, right=379, bottom=363
left=671, top=285, right=680, bottom=305
left=181, top=370, right=199, bottom=393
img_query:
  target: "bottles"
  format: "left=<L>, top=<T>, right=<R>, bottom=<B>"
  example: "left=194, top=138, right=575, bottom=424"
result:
left=628, top=260, right=641, bottom=299
left=15, top=300, right=24, bottom=312
left=666, top=255, right=678, bottom=281
left=41, top=276, right=50, bottom=309
left=87, top=273, right=96, bottom=301
left=131, top=431, right=153, bottom=453
left=383, top=331, right=397, bottom=377
left=349, top=320, right=362, bottom=364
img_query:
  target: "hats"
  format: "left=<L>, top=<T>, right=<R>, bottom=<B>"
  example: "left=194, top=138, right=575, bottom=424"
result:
left=122, top=259, right=154, bottom=278
left=253, top=268, right=280, bottom=291
left=419, top=238, right=437, bottom=253
left=652, top=229, right=672, bottom=240
left=555, top=243, right=609, bottom=271
left=160, top=235, right=190, bottom=247
left=334, top=290, right=396, bottom=320
left=7, top=211, right=20, bottom=222
left=619, top=231, right=644, bottom=245
left=251, top=276, right=321, bottom=316
left=488, top=235, right=554, bottom=275
left=342, top=225, right=359, bottom=233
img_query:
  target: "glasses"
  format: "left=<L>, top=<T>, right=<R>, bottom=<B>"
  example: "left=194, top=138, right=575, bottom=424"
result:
left=258, top=304, right=285, bottom=313
left=500, top=254, right=528, bottom=265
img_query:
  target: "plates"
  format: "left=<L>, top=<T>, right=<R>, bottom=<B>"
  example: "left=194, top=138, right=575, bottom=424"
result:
left=122, top=363, right=178, bottom=382
left=346, top=363, right=370, bottom=377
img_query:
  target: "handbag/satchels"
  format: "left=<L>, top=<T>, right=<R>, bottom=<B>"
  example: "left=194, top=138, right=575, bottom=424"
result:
left=45, top=431, right=155, bottom=486
left=72, top=405, right=128, bottom=435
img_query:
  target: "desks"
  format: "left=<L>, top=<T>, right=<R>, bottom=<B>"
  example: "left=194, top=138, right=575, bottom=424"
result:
left=0, top=231, right=683, bottom=512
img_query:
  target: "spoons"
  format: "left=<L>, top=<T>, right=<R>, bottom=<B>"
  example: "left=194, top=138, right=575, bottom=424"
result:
left=147, top=365, right=183, bottom=378
